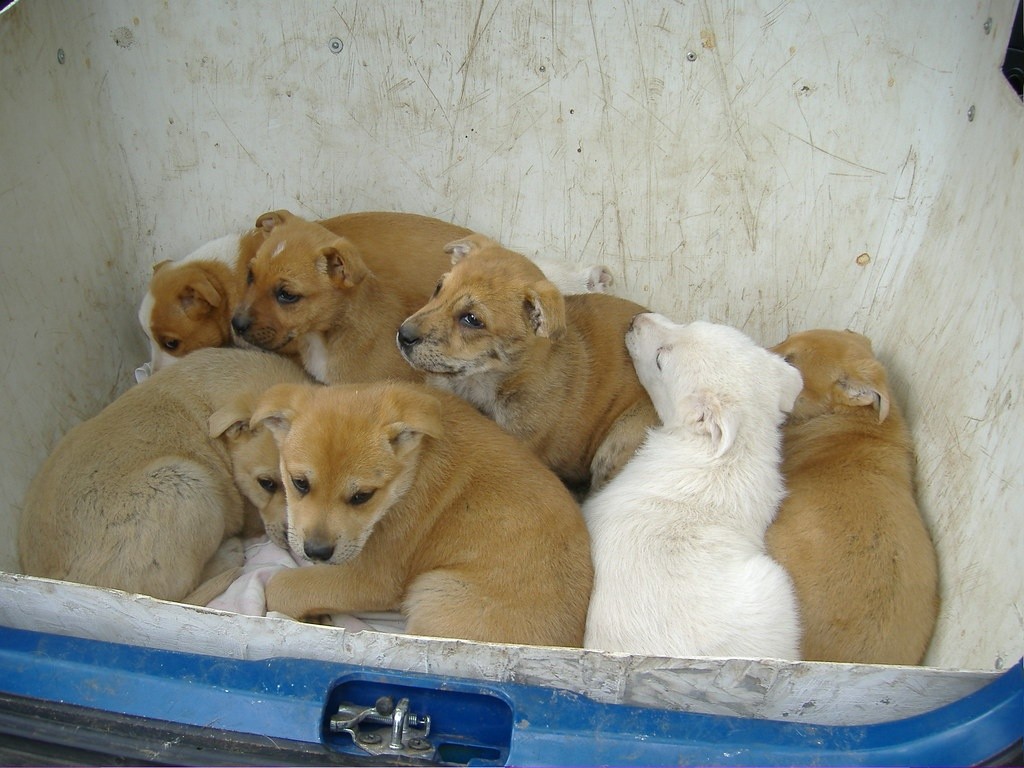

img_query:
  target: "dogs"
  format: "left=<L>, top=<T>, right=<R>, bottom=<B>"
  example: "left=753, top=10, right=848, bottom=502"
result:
left=16, top=210, right=939, bottom=666
